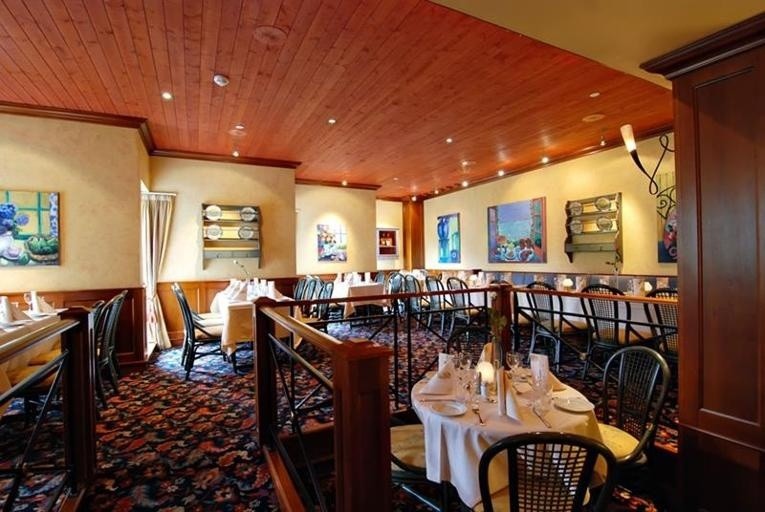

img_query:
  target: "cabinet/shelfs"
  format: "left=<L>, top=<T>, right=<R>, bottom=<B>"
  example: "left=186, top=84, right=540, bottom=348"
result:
left=376, top=226, right=399, bottom=260
left=563, top=192, right=623, bottom=263
left=201, top=204, right=262, bottom=269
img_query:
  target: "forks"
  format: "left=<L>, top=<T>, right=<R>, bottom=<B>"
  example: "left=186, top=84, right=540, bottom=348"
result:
left=471, top=404, right=486, bottom=426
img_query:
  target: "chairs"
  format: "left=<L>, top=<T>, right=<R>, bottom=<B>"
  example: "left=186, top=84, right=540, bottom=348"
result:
left=291, top=278, right=334, bottom=356
left=171, top=283, right=238, bottom=380
left=11, top=288, right=129, bottom=445
left=473, top=431, right=617, bottom=512
left=389, top=425, right=449, bottom=512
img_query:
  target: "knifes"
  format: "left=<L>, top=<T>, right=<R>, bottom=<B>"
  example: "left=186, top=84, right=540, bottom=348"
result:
left=532, top=408, right=551, bottom=429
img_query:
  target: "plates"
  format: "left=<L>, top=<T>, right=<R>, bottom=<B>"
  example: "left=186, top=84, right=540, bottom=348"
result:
left=554, top=397, right=595, bottom=413
left=240, top=207, right=256, bottom=222
left=568, top=202, right=583, bottom=216
left=430, top=401, right=467, bottom=418
left=596, top=216, right=612, bottom=232
left=425, top=370, right=437, bottom=379
left=595, top=195, right=611, bottom=211
left=237, top=225, right=254, bottom=239
left=568, top=220, right=584, bottom=234
left=205, top=205, right=222, bottom=221
left=207, top=223, right=221, bottom=240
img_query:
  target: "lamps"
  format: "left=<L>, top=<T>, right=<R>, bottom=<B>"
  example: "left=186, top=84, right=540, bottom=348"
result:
left=621, top=125, right=674, bottom=193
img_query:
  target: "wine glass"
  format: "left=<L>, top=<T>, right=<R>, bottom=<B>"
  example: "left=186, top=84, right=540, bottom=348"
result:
left=451, top=351, right=553, bottom=414
